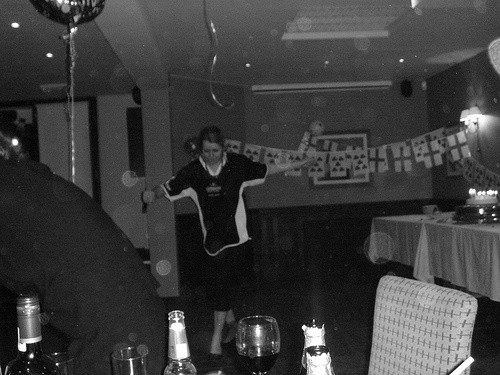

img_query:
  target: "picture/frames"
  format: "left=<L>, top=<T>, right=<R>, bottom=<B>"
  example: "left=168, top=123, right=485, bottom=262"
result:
left=310, top=130, right=374, bottom=186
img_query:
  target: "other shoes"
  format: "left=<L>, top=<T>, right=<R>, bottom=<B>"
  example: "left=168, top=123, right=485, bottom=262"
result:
left=221, top=337, right=235, bottom=348
left=190, top=350, right=223, bottom=365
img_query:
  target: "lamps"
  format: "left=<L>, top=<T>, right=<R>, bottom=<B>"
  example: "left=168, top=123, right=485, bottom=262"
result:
left=459, top=104, right=483, bottom=152
left=252, top=80, right=393, bottom=94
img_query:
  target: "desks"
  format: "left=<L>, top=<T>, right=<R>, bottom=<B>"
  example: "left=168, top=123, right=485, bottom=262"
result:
left=368, top=211, right=500, bottom=331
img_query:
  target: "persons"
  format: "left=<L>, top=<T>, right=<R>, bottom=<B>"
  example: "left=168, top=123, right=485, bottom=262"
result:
left=142, top=125, right=316, bottom=362
left=0, top=133, right=169, bottom=375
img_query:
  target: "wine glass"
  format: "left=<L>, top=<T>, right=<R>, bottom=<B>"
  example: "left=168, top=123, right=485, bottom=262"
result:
left=235, top=315, right=281, bottom=375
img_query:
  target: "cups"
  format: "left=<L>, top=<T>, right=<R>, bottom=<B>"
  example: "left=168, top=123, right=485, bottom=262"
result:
left=45, top=353, right=81, bottom=375
left=111, top=346, right=150, bottom=375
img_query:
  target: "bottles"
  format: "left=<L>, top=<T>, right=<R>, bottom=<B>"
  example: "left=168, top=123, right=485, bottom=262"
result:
left=5, top=294, right=61, bottom=375
left=299, top=319, right=326, bottom=375
left=164, top=310, right=197, bottom=375
left=305, top=345, right=335, bottom=375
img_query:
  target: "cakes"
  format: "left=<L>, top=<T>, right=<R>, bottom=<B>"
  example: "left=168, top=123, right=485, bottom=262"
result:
left=466, top=196, right=499, bottom=205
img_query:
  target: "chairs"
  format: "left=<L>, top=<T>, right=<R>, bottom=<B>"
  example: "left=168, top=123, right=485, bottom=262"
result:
left=367, top=275, right=479, bottom=375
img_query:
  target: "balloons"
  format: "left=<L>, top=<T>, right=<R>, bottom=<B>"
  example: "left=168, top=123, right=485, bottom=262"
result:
left=30, top=0, right=105, bottom=26
